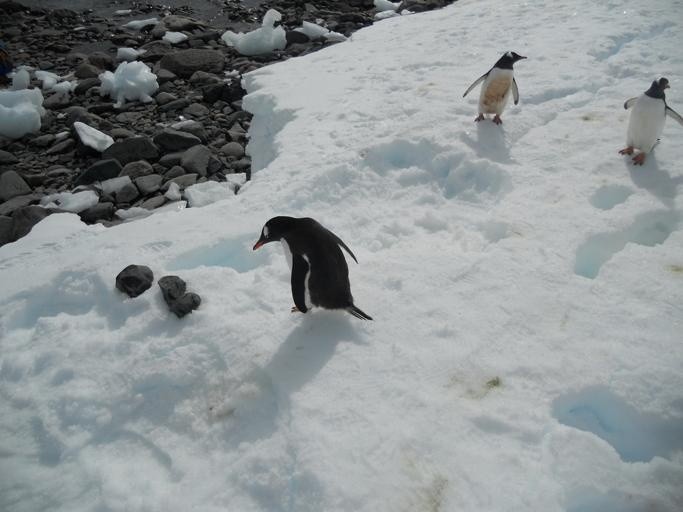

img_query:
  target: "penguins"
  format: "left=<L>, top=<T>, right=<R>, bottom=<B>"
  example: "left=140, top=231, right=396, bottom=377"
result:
left=462, top=51, right=528, bottom=124
left=253, top=215, right=374, bottom=322
left=619, top=76, right=682, bottom=166
